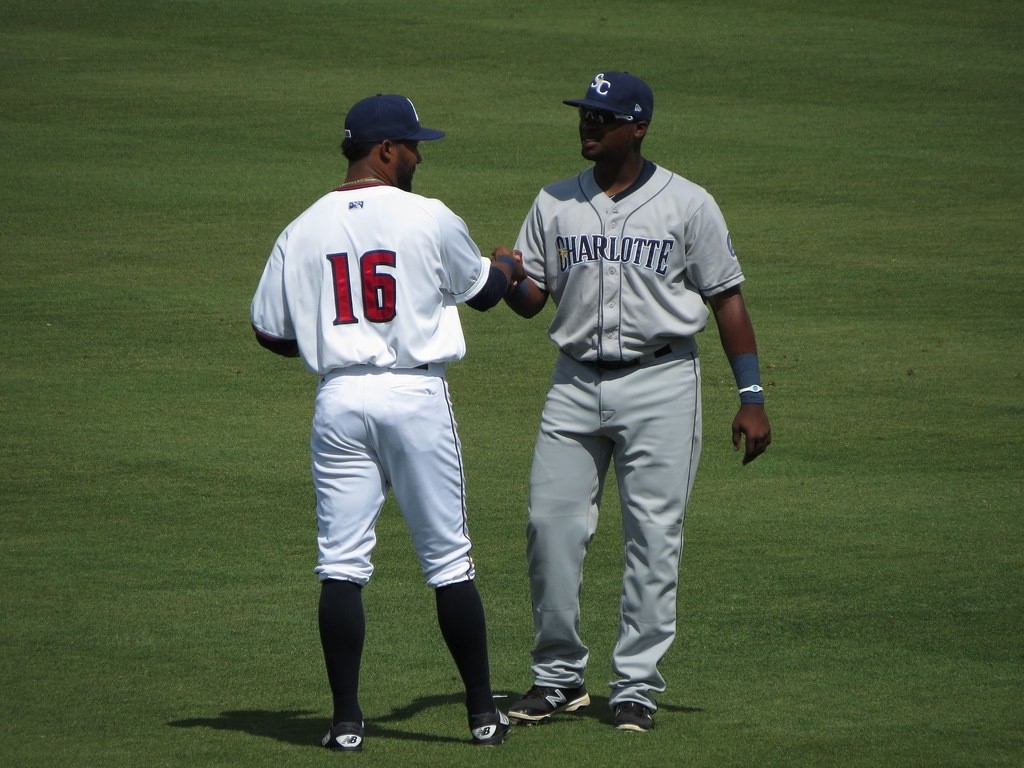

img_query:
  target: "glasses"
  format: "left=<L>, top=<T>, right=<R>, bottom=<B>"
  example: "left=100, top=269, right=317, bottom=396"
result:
left=579, top=104, right=641, bottom=127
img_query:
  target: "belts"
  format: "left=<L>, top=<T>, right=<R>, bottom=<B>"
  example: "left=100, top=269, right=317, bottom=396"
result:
left=417, top=363, right=431, bottom=371
left=581, top=345, right=670, bottom=371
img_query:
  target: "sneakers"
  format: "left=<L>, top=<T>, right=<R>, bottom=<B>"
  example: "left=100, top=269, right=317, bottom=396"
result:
left=507, top=682, right=590, bottom=721
left=614, top=700, right=654, bottom=736
left=321, top=718, right=365, bottom=752
left=469, top=707, right=511, bottom=746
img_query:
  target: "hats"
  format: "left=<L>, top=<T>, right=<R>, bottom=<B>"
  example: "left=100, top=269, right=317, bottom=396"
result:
left=561, top=71, right=653, bottom=123
left=345, top=94, right=445, bottom=142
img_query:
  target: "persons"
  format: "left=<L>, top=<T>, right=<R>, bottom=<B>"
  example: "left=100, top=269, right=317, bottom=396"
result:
left=497, top=70, right=771, bottom=731
left=249, top=93, right=527, bottom=754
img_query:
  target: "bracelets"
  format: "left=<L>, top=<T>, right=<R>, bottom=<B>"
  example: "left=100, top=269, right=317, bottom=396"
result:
left=510, top=271, right=528, bottom=289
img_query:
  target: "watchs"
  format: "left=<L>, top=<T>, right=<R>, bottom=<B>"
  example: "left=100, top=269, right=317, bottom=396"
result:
left=738, top=384, right=764, bottom=395
